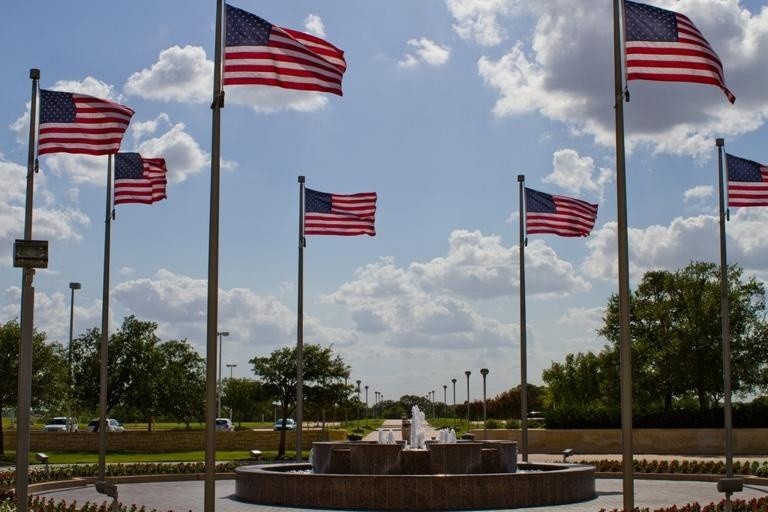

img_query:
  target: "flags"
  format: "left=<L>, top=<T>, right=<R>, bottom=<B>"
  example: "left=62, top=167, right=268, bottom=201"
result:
left=725, top=152, right=768, bottom=209
left=37, top=89, right=136, bottom=157
left=621, top=1, right=736, bottom=105
left=112, top=149, right=169, bottom=205
left=303, top=185, right=378, bottom=237
left=222, top=2, right=348, bottom=99
left=524, top=187, right=600, bottom=239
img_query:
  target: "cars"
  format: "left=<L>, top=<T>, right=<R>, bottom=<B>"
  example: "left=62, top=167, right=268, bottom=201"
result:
left=274, top=418, right=297, bottom=430
left=213, top=418, right=233, bottom=431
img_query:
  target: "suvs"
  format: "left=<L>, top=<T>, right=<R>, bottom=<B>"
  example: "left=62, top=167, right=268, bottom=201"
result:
left=526, top=411, right=545, bottom=423
left=43, top=416, right=79, bottom=433
left=88, top=417, right=124, bottom=433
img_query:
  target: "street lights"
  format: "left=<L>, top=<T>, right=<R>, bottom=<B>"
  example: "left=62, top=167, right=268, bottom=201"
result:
left=462, top=371, right=474, bottom=430
left=374, top=392, right=384, bottom=418
left=479, top=368, right=490, bottom=438
left=342, top=372, right=351, bottom=433
left=214, top=331, right=229, bottom=421
left=224, top=360, right=238, bottom=434
left=354, top=379, right=364, bottom=433
left=364, top=385, right=369, bottom=423
left=442, top=385, right=448, bottom=423
left=451, top=379, right=460, bottom=423
left=425, top=390, right=437, bottom=420
